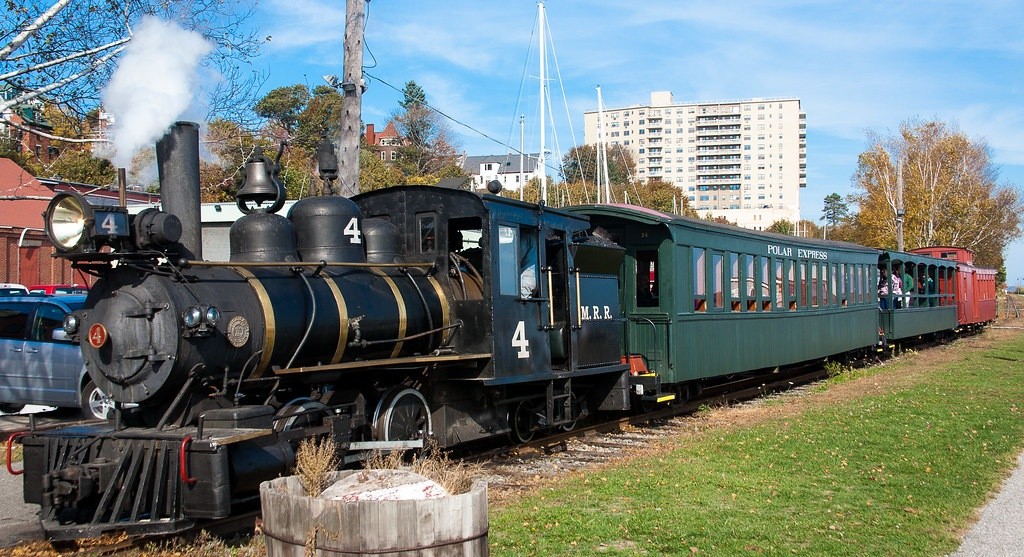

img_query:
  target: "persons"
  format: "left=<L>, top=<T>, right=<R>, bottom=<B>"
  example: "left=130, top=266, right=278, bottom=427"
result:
left=897, top=267, right=913, bottom=307
left=517, top=223, right=538, bottom=299
left=893, top=269, right=935, bottom=308
left=878, top=266, right=899, bottom=309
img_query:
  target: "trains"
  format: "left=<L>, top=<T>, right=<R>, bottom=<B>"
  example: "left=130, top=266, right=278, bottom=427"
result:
left=6, top=122, right=999, bottom=554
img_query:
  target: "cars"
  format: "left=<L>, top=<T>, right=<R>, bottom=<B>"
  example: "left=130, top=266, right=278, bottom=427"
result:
left=0, top=284, right=29, bottom=295
left=27, top=284, right=91, bottom=295
left=0, top=295, right=114, bottom=421
